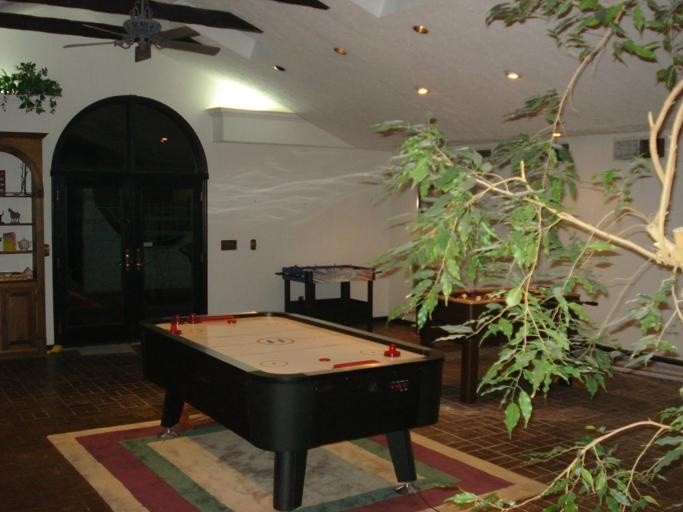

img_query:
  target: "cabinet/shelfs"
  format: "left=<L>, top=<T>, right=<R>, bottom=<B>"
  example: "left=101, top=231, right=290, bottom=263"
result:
left=0, top=127, right=49, bottom=364
left=279, top=262, right=378, bottom=333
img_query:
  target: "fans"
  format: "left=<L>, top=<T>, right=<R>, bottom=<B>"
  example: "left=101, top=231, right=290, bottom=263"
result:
left=62, top=15, right=220, bottom=62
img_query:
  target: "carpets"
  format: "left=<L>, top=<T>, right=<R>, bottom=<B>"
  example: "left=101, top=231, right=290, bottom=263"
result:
left=45, top=412, right=550, bottom=512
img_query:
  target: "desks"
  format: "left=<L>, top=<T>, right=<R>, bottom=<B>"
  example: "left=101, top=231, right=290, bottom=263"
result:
left=413, top=290, right=581, bottom=403
left=138, top=308, right=448, bottom=511
